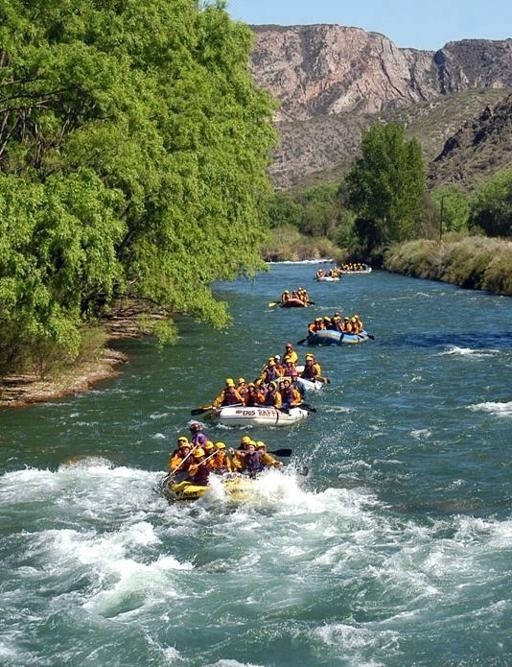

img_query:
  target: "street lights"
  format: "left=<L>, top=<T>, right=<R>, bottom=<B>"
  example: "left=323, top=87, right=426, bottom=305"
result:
left=439, top=193, right=452, bottom=241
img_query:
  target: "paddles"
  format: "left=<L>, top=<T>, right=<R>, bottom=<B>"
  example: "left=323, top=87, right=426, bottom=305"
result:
left=234, top=447, right=292, bottom=457
left=359, top=330, right=374, bottom=340
left=174, top=449, right=221, bottom=482
left=190, top=403, right=242, bottom=415
left=298, top=333, right=310, bottom=345
left=268, top=301, right=280, bottom=306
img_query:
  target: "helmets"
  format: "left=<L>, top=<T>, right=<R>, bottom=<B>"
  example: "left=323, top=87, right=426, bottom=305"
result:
left=224, top=342, right=315, bottom=390
left=316, top=312, right=359, bottom=322
left=283, top=287, right=306, bottom=296
left=318, top=263, right=364, bottom=272
left=177, top=423, right=266, bottom=458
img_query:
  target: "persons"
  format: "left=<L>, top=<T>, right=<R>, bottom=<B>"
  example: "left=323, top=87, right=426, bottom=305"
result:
left=177, top=423, right=281, bottom=485
left=215, top=378, right=302, bottom=411
left=342, top=262, right=364, bottom=271
left=309, top=312, right=362, bottom=335
left=260, top=343, right=321, bottom=384
left=282, top=287, right=308, bottom=307
left=317, top=268, right=340, bottom=277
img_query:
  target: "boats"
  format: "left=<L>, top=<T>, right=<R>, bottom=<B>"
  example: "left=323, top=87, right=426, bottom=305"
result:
left=337, top=263, right=372, bottom=274
left=164, top=469, right=280, bottom=500
left=279, top=298, right=307, bottom=307
left=317, top=275, right=340, bottom=282
left=307, top=327, right=369, bottom=344
left=210, top=401, right=309, bottom=426
left=269, top=364, right=324, bottom=392
